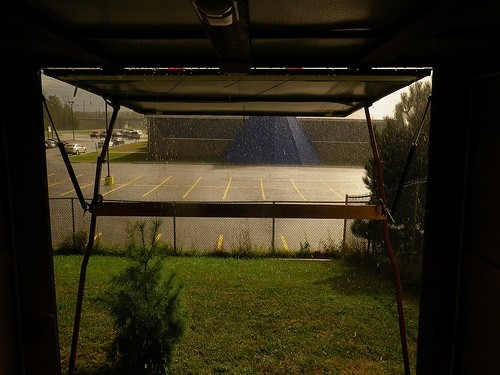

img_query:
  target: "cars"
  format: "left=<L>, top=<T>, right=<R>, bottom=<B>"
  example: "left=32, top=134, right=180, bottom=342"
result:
left=45, top=138, right=59, bottom=148
left=89, top=130, right=140, bottom=139
left=112, top=137, right=125, bottom=145
left=97, top=139, right=113, bottom=148
left=64, top=144, right=87, bottom=155
